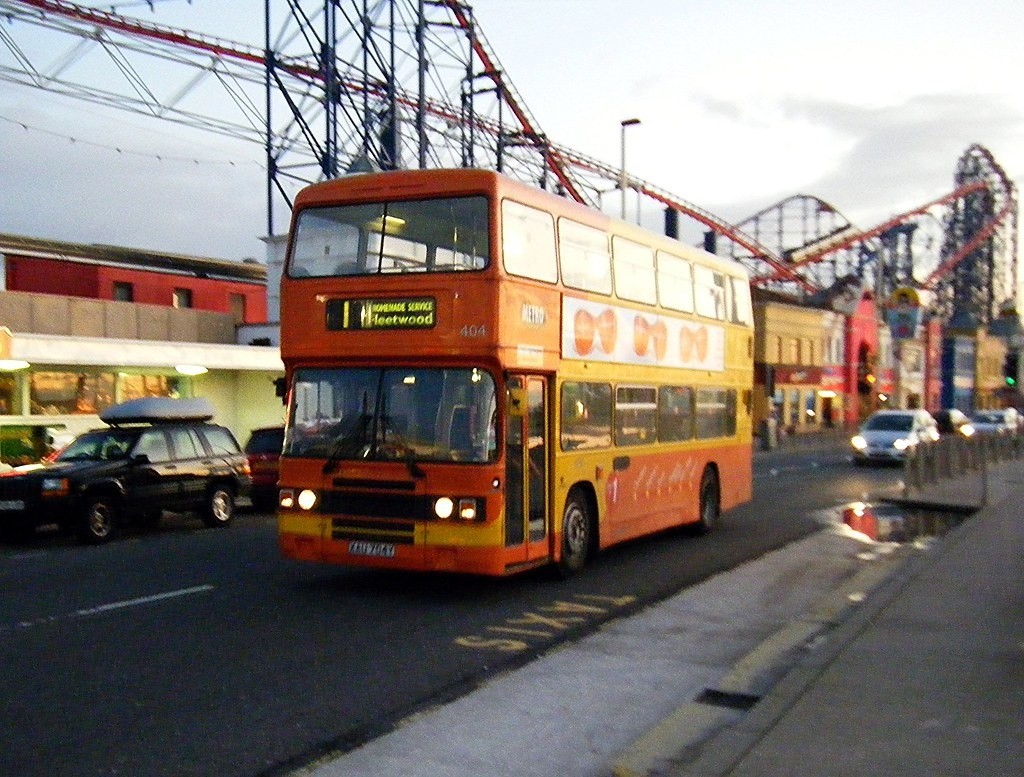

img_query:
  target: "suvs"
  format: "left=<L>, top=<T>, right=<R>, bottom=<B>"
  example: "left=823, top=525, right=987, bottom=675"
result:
left=0, top=395, right=251, bottom=544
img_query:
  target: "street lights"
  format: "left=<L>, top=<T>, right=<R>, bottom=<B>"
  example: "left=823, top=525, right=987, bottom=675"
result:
left=619, top=117, right=643, bottom=218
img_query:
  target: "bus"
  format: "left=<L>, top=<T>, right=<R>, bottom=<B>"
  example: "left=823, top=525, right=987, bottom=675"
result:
left=278, top=166, right=756, bottom=578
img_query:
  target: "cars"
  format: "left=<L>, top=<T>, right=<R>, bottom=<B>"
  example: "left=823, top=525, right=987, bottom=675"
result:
left=851, top=408, right=940, bottom=467
left=931, top=408, right=1024, bottom=445
left=246, top=424, right=290, bottom=510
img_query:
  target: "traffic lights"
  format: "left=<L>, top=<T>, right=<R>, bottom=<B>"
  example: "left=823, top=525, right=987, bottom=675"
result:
left=1003, top=354, right=1018, bottom=386
left=858, top=366, right=877, bottom=395
